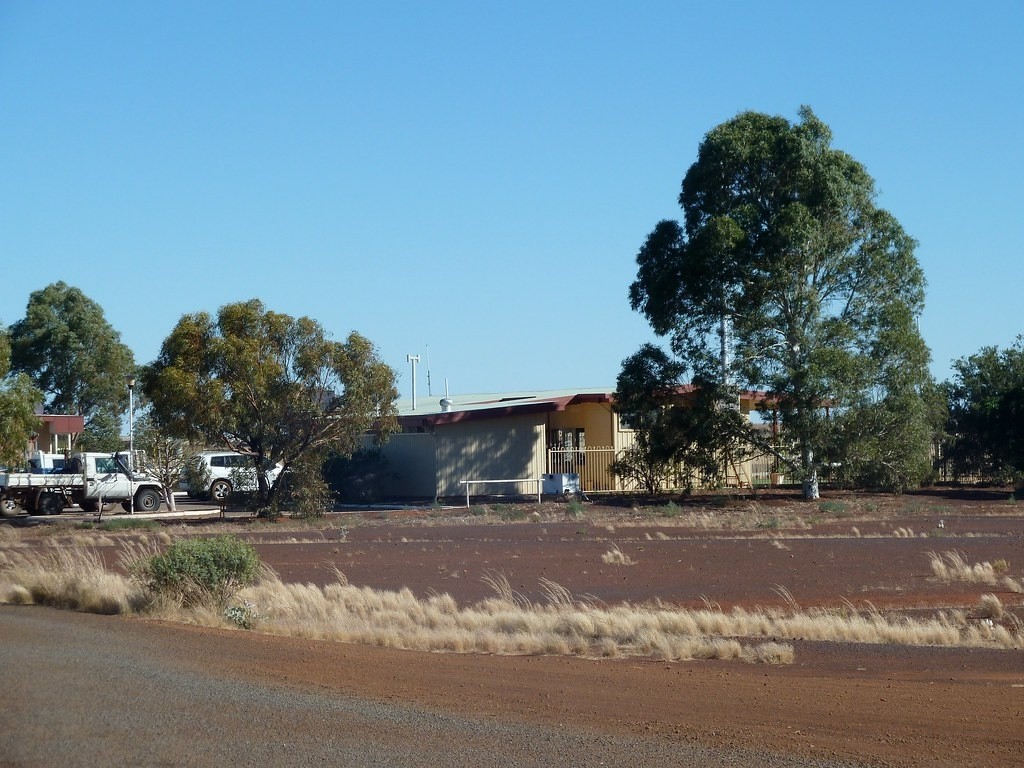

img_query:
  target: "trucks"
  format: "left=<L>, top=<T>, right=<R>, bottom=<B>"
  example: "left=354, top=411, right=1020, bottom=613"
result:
left=0, top=447, right=169, bottom=520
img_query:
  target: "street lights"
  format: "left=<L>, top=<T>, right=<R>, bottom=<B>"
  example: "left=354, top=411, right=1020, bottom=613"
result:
left=124, top=370, right=137, bottom=516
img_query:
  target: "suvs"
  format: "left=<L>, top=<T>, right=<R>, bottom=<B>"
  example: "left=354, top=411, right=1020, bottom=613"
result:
left=179, top=447, right=292, bottom=504
